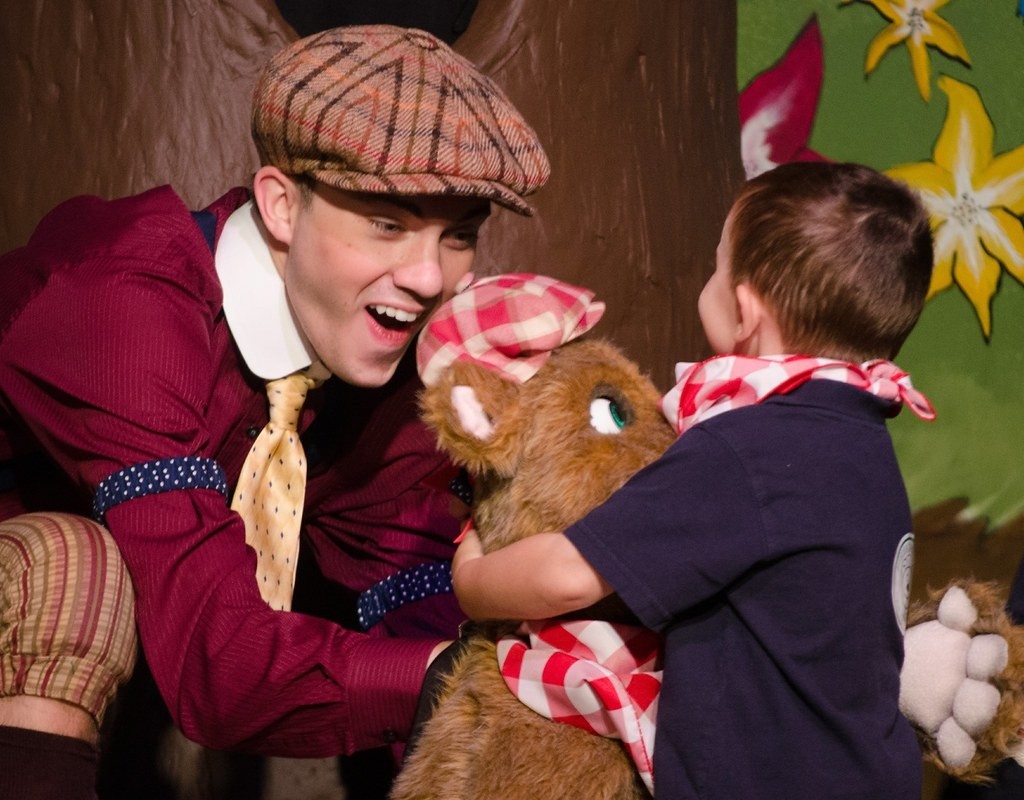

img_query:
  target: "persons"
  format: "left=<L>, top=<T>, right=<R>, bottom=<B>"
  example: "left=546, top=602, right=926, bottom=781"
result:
left=446, top=160, right=939, bottom=800
left=0, top=23, right=550, bottom=800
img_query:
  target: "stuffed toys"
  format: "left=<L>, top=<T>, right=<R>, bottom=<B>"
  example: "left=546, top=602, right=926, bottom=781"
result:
left=391, top=268, right=1024, bottom=800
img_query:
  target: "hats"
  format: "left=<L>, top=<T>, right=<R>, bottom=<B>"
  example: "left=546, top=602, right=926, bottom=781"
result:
left=249, top=25, right=552, bottom=218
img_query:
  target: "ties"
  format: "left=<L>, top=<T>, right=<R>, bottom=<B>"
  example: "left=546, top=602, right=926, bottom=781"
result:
left=229, top=359, right=321, bottom=612
